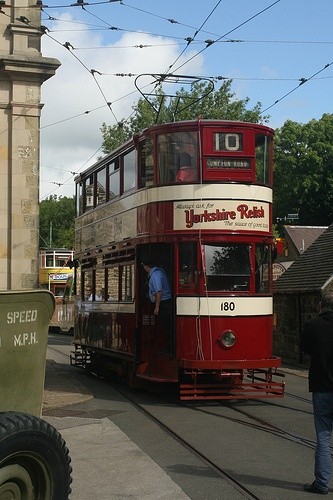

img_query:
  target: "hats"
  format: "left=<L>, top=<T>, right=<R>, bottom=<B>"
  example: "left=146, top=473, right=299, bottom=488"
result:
left=140, top=256, right=155, bottom=265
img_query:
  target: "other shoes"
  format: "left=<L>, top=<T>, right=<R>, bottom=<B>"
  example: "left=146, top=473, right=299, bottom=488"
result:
left=302, top=481, right=328, bottom=494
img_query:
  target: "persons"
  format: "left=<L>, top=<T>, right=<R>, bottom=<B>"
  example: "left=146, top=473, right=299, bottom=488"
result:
left=141, top=256, right=175, bottom=358
left=85, top=286, right=116, bottom=346
left=299, top=296, right=333, bottom=495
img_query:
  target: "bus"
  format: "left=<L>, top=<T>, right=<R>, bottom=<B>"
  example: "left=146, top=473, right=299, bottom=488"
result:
left=37, top=220, right=75, bottom=334
left=73, top=72, right=286, bottom=401
